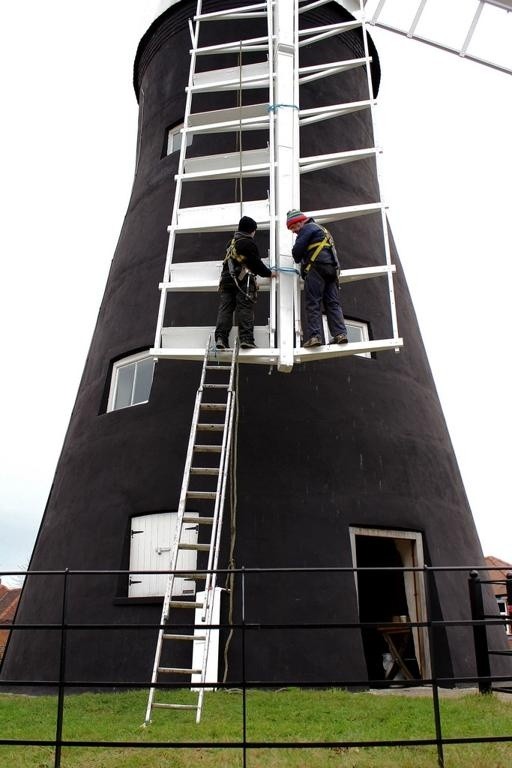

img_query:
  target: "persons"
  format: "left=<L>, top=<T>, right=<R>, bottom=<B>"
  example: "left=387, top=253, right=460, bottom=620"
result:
left=214, top=215, right=278, bottom=350
left=287, top=209, right=348, bottom=348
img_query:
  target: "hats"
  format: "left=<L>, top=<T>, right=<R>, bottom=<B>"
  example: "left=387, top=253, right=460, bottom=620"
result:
left=287, top=208, right=307, bottom=227
left=238, top=215, right=257, bottom=233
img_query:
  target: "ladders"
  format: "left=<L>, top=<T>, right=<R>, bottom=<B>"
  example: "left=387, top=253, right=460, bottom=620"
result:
left=164, top=333, right=239, bottom=622
left=144, top=386, right=235, bottom=726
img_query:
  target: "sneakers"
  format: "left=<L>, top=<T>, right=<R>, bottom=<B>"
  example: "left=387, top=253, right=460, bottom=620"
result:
left=216, top=333, right=348, bottom=347
